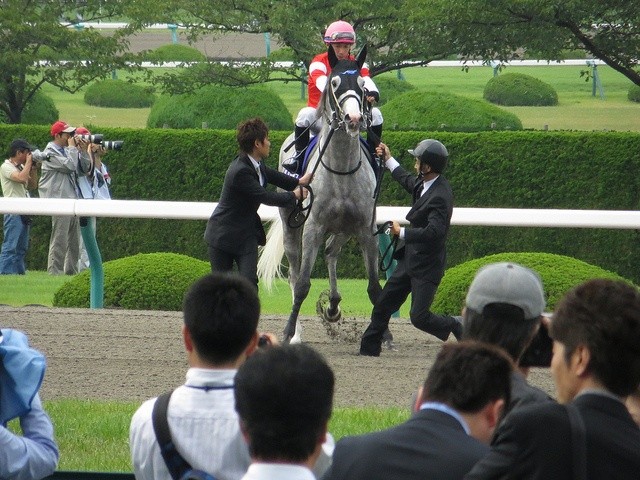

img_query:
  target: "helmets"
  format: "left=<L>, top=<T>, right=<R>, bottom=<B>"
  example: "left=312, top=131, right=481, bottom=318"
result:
left=324, top=20, right=356, bottom=47
left=406, top=138, right=449, bottom=173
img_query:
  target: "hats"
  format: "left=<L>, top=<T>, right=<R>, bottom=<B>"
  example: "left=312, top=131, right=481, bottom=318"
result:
left=75, top=127, right=91, bottom=135
left=467, top=262, right=545, bottom=320
left=51, top=121, right=76, bottom=136
left=9, top=138, right=39, bottom=150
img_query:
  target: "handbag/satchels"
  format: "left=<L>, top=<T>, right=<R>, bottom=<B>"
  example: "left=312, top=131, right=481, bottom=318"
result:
left=20, top=216, right=37, bottom=224
left=79, top=216, right=87, bottom=226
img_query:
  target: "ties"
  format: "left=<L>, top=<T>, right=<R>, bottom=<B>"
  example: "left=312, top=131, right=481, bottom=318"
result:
left=256, top=166, right=261, bottom=174
left=415, top=184, right=424, bottom=201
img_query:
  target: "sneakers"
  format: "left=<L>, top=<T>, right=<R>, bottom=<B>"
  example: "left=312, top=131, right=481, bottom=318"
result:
left=452, top=315, right=464, bottom=343
left=363, top=342, right=380, bottom=356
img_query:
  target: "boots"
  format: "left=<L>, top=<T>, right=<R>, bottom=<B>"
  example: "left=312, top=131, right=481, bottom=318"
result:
left=366, top=124, right=382, bottom=171
left=281, top=123, right=309, bottom=175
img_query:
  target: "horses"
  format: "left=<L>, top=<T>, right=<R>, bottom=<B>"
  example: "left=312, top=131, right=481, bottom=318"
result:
left=256, top=41, right=399, bottom=356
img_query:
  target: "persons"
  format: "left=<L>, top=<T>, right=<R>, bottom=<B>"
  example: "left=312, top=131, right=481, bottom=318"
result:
left=202, top=116, right=314, bottom=298
left=0, top=138, right=38, bottom=274
left=329, top=338, right=513, bottom=480
left=279, top=19, right=384, bottom=176
left=128, top=270, right=337, bottom=479
left=460, top=279, right=640, bottom=478
left=0, top=329, right=60, bottom=477
left=231, top=341, right=335, bottom=479
left=66, top=126, right=106, bottom=271
left=37, top=120, right=91, bottom=276
left=460, top=261, right=559, bottom=444
left=358, top=135, right=465, bottom=354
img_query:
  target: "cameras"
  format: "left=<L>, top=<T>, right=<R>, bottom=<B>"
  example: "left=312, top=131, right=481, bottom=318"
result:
left=72, top=130, right=107, bottom=147
left=257, top=334, right=273, bottom=351
left=27, top=150, right=53, bottom=166
left=518, top=321, right=563, bottom=370
left=102, top=139, right=126, bottom=156
